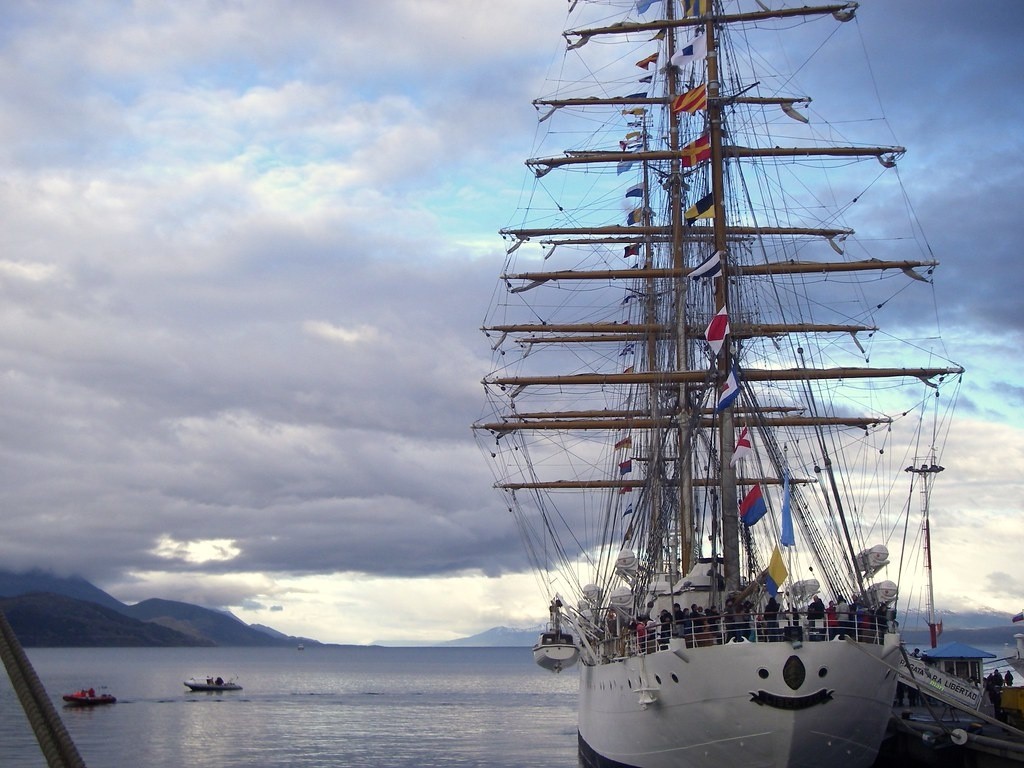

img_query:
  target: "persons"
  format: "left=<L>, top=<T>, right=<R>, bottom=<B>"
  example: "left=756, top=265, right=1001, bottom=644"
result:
left=611, top=593, right=1015, bottom=721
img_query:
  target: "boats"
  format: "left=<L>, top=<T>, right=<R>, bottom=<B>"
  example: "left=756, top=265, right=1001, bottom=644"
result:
left=532, top=596, right=580, bottom=673
left=298, top=644, right=304, bottom=650
left=184, top=675, right=243, bottom=691
left=63, top=688, right=116, bottom=704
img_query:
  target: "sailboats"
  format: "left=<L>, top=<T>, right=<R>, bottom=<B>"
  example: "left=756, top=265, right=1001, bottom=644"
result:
left=470, top=0, right=965, bottom=768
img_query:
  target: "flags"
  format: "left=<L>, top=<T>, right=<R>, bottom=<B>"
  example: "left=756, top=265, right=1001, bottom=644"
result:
left=672, top=83, right=708, bottom=113
left=718, top=367, right=742, bottom=411
left=681, top=135, right=710, bottom=167
left=613, top=1, right=668, bottom=519
left=682, top=0, right=712, bottom=18
left=739, top=482, right=768, bottom=527
left=728, top=425, right=754, bottom=466
left=763, top=545, right=789, bottom=596
left=780, top=465, right=796, bottom=545
left=687, top=250, right=723, bottom=278
left=670, top=32, right=707, bottom=66
left=684, top=192, right=716, bottom=225
left=703, top=305, right=731, bottom=356
